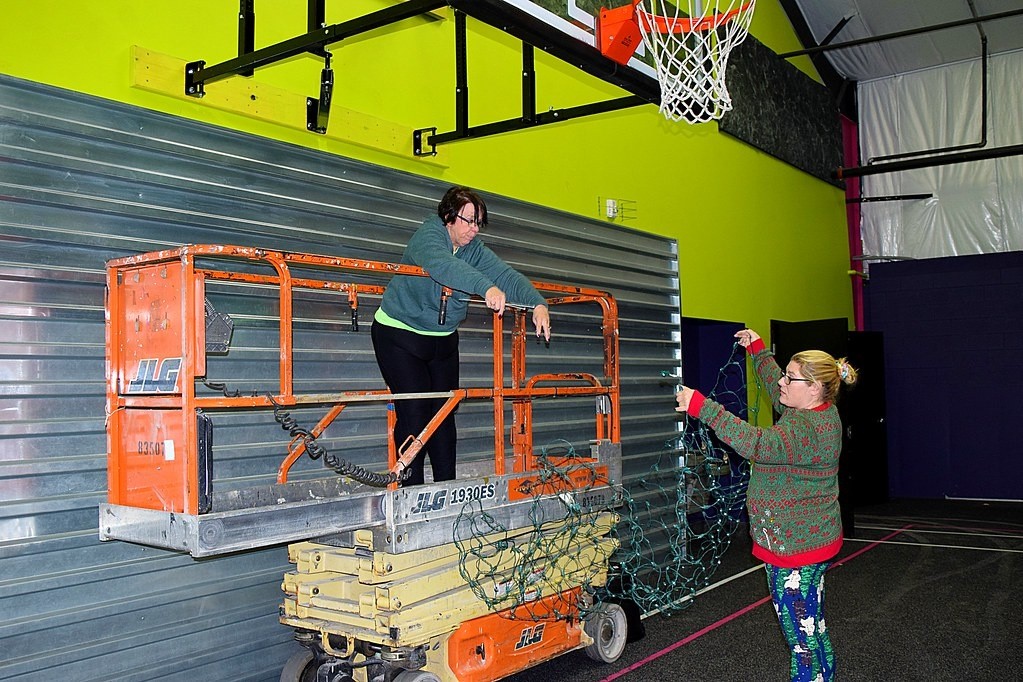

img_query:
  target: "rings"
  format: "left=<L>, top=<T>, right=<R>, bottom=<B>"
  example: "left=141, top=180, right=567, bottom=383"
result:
left=537, top=325, right=541, bottom=329
left=548, top=327, right=552, bottom=329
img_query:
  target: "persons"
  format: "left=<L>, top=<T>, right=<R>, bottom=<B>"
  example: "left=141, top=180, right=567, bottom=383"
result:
left=674, top=328, right=858, bottom=682
left=371, top=185, right=550, bottom=490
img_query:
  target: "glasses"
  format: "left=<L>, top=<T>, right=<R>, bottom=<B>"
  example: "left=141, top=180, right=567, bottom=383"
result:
left=781, top=371, right=811, bottom=385
left=457, top=214, right=484, bottom=228
